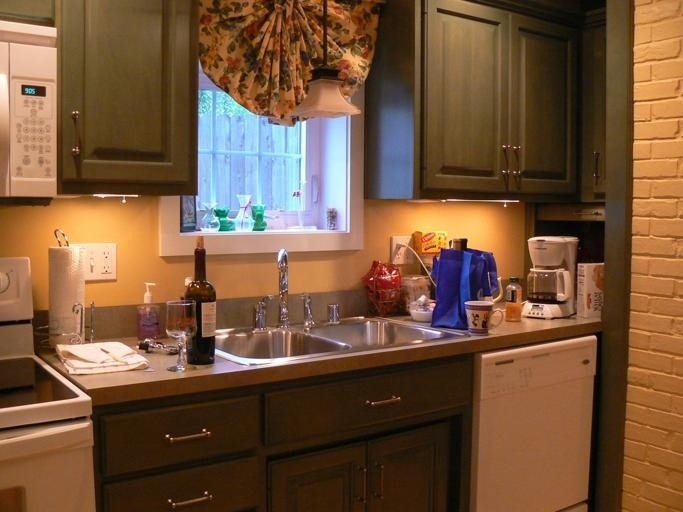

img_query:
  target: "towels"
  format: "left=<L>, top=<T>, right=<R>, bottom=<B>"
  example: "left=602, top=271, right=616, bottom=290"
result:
left=54, top=339, right=154, bottom=376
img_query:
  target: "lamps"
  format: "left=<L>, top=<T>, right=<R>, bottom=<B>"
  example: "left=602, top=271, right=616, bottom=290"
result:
left=289, top=0, right=362, bottom=119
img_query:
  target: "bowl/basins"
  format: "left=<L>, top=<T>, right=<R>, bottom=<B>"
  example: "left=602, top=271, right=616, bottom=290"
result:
left=407, top=308, right=431, bottom=323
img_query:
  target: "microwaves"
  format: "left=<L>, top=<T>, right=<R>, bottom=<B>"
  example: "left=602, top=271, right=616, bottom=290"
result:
left=0, top=19, right=59, bottom=200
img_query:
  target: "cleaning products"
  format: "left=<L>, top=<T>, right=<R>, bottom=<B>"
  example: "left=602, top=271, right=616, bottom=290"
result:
left=136, top=282, right=161, bottom=340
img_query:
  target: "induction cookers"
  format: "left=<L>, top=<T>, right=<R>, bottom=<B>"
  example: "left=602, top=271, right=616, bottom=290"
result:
left=0, top=356, right=91, bottom=411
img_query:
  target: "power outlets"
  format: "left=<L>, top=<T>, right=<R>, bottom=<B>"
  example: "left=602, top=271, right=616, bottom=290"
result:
left=389, top=235, right=415, bottom=265
left=80, top=242, right=116, bottom=282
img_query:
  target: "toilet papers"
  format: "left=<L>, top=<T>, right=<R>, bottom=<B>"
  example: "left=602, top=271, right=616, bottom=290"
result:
left=47, top=246, right=85, bottom=348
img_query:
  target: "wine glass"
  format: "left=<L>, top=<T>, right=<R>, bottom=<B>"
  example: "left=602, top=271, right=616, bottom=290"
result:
left=164, top=300, right=198, bottom=373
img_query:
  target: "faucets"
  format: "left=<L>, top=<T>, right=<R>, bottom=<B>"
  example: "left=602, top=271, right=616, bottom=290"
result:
left=276, top=249, right=291, bottom=327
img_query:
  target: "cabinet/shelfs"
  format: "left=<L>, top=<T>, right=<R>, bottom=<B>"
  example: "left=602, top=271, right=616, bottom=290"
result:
left=363, top=1, right=580, bottom=205
left=265, top=353, right=472, bottom=511
left=579, top=7, right=606, bottom=203
left=55, top=0, right=198, bottom=197
left=93, top=389, right=265, bottom=511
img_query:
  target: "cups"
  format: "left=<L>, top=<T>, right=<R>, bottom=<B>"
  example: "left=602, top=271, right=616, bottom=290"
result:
left=463, top=299, right=504, bottom=335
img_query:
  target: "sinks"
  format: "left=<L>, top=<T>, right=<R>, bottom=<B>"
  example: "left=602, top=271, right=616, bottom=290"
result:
left=214, top=326, right=351, bottom=367
left=302, top=316, right=467, bottom=358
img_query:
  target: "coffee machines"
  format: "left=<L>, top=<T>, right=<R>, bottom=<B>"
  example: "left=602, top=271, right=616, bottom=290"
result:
left=522, top=236, right=579, bottom=321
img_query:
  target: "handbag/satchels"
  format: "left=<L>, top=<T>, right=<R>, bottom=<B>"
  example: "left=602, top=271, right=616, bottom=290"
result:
left=431, top=248, right=499, bottom=330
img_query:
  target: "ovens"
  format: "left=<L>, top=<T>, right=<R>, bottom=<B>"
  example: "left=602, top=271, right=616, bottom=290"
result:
left=0, top=418, right=98, bottom=512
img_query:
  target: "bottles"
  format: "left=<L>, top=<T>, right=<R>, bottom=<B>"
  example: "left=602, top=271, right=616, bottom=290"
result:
left=199, top=195, right=269, bottom=232
left=185, top=235, right=216, bottom=365
left=326, top=208, right=336, bottom=230
left=504, top=277, right=522, bottom=321
